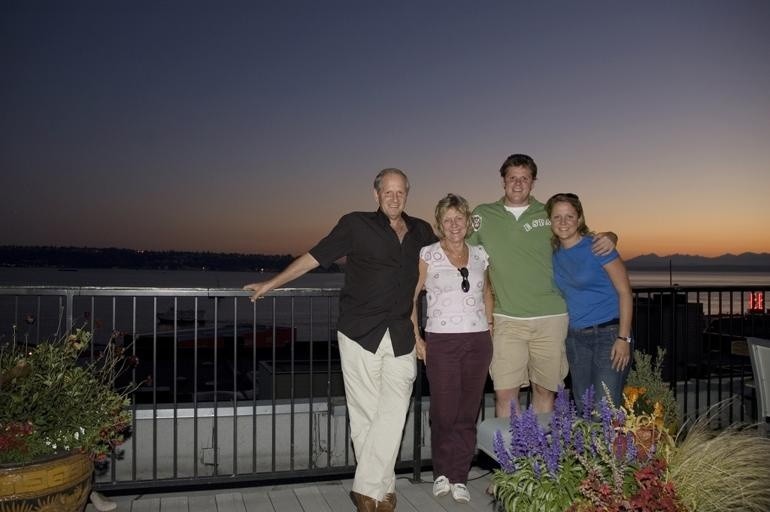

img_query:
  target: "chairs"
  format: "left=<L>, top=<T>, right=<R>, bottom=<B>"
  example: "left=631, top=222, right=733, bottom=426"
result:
left=747, top=337, right=770, bottom=442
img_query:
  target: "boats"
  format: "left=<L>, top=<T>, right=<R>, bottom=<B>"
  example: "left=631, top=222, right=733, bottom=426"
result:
left=124, top=305, right=303, bottom=370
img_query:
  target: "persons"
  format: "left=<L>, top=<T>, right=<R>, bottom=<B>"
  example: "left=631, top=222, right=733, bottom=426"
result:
left=242, top=169, right=437, bottom=512
left=468, top=153, right=618, bottom=416
left=545, top=192, right=632, bottom=422
left=410, top=194, right=495, bottom=502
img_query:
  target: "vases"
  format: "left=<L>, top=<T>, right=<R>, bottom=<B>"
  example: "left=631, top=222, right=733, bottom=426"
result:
left=0, top=455, right=96, bottom=512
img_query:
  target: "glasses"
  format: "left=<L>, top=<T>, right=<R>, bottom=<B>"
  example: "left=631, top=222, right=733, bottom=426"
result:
left=458, top=267, right=470, bottom=292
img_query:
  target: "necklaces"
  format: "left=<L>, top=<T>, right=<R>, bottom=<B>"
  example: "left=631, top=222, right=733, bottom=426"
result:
left=445, top=241, right=466, bottom=259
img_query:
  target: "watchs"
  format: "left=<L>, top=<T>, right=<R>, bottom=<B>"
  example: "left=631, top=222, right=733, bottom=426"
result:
left=618, top=336, right=631, bottom=343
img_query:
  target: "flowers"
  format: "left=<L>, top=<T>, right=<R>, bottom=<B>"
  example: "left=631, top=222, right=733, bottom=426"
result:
left=0, top=316, right=153, bottom=465
left=487, top=345, right=690, bottom=512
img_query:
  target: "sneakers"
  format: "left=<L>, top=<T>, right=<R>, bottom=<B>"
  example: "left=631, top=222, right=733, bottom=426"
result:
left=432, top=475, right=470, bottom=503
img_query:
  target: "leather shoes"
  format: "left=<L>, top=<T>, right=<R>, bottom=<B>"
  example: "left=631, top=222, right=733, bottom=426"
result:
left=350, top=491, right=397, bottom=512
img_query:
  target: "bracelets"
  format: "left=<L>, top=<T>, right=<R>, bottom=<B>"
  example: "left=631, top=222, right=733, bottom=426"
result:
left=488, top=322, right=492, bottom=325
